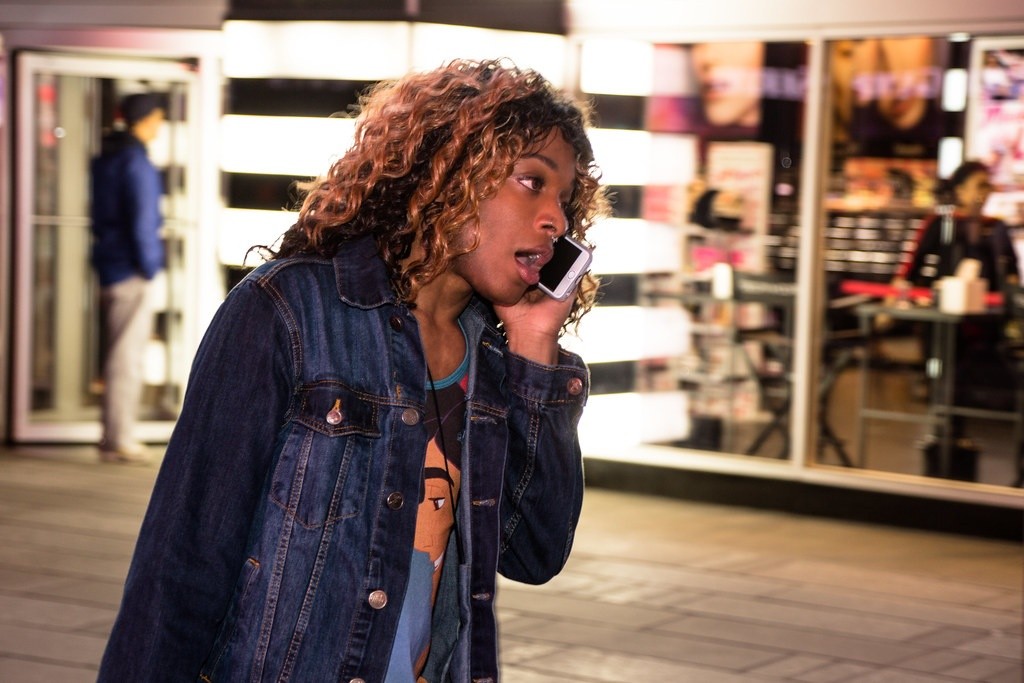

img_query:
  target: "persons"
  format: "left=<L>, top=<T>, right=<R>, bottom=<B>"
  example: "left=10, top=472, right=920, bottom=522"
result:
left=874, top=161, right=1018, bottom=330
left=97, top=60, right=612, bottom=683
left=90, top=91, right=164, bottom=465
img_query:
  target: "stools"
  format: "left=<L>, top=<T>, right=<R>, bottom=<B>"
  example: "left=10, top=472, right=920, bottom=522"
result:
left=731, top=322, right=867, bottom=467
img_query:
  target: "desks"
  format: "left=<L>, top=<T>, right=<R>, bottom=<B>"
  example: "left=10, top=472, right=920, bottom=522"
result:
left=863, top=294, right=1011, bottom=474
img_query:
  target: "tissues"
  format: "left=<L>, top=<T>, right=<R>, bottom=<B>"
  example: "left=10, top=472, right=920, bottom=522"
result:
left=938, top=257, right=989, bottom=315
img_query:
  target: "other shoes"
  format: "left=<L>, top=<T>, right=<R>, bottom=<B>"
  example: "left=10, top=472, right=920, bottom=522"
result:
left=98, top=437, right=152, bottom=464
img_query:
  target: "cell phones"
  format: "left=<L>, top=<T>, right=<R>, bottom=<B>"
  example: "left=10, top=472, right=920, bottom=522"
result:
left=536, top=235, right=592, bottom=302
